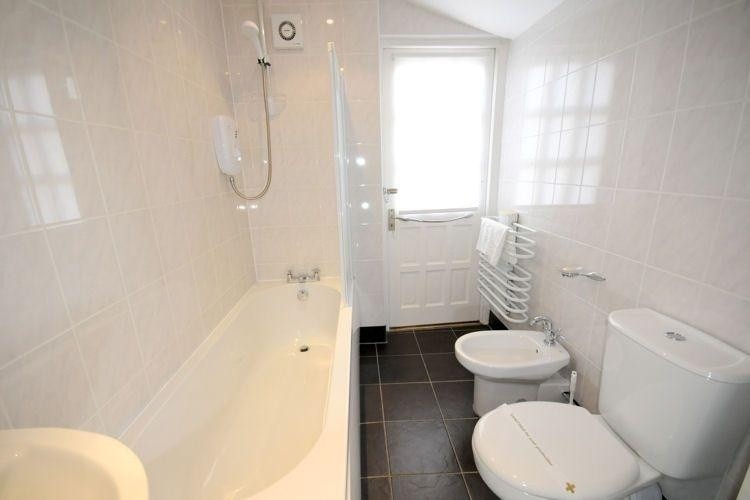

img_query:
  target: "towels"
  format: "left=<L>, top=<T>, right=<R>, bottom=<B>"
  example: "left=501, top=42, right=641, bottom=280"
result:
left=476, top=217, right=515, bottom=266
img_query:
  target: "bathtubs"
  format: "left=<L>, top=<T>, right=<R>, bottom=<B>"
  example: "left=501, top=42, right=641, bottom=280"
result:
left=116, top=274, right=364, bottom=500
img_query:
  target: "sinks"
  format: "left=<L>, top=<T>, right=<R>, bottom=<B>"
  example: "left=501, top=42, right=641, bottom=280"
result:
left=1, top=426, right=151, bottom=500
left=453, top=329, right=570, bottom=382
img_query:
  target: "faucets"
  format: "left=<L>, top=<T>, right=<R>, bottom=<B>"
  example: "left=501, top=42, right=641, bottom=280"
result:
left=298, top=274, right=311, bottom=283
left=529, top=315, right=560, bottom=344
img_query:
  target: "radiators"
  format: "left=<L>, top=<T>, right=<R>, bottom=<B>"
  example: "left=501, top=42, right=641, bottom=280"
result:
left=470, top=220, right=538, bottom=324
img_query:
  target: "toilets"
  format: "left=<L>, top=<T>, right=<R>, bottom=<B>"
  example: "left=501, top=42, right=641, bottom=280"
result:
left=469, top=307, right=750, bottom=500
